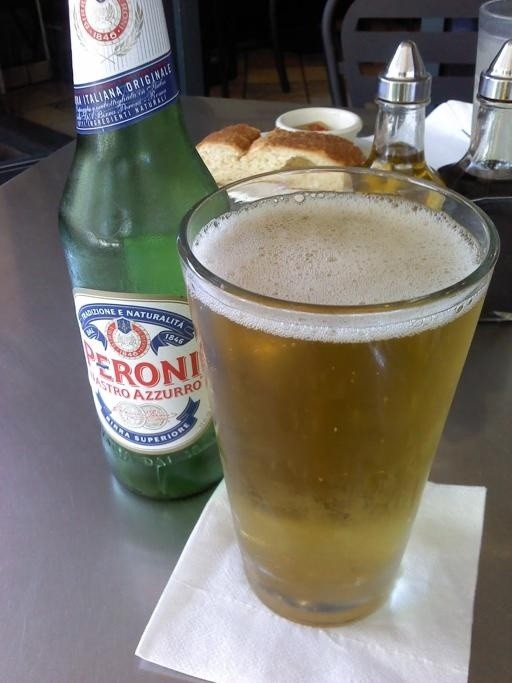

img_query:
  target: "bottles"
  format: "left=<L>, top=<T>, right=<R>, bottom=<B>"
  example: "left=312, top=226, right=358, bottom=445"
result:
left=55, top=0, right=234, bottom=505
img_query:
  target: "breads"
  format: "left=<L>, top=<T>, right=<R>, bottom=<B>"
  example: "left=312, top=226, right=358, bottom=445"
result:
left=197, top=124, right=366, bottom=193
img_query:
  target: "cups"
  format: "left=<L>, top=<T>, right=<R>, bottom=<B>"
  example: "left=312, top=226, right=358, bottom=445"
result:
left=275, top=108, right=362, bottom=147
left=177, top=163, right=501, bottom=631
